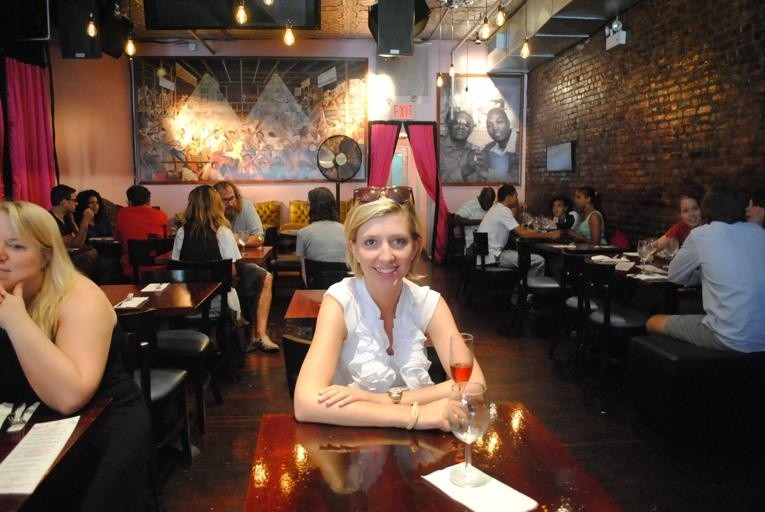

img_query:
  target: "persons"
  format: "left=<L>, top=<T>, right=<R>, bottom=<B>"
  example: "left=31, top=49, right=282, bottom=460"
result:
left=440, top=110, right=480, bottom=181
left=212, top=180, right=280, bottom=355
left=76, top=188, right=113, bottom=237
left=292, top=184, right=488, bottom=434
left=648, top=193, right=710, bottom=255
left=113, top=184, right=172, bottom=283
left=453, top=183, right=608, bottom=304
left=0, top=199, right=154, bottom=512
left=169, top=184, right=242, bottom=325
left=644, top=183, right=765, bottom=358
left=743, top=189, right=765, bottom=229
left=476, top=108, right=519, bottom=182
left=48, top=184, right=99, bottom=276
left=295, top=186, right=351, bottom=289
left=173, top=125, right=322, bottom=181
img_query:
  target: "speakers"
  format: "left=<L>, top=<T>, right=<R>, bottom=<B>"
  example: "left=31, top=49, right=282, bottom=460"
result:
left=57, top=0, right=103, bottom=60
left=367, top=0, right=432, bottom=44
left=102, top=8, right=134, bottom=60
left=377, top=0, right=415, bottom=59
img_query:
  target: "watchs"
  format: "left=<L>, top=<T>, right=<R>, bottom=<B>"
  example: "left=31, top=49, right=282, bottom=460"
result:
left=387, top=386, right=403, bottom=404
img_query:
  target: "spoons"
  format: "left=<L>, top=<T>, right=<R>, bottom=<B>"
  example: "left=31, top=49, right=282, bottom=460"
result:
left=117, top=293, right=134, bottom=307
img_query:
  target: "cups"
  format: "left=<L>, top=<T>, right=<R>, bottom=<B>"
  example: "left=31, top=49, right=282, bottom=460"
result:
left=85, top=208, right=93, bottom=225
left=524, top=216, right=549, bottom=232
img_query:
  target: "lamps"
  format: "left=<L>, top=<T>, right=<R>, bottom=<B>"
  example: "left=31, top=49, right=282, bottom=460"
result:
left=605, top=21, right=626, bottom=51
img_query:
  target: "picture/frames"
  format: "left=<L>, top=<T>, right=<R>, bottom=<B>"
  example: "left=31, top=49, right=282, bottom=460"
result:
left=127, top=55, right=370, bottom=186
left=436, top=73, right=526, bottom=186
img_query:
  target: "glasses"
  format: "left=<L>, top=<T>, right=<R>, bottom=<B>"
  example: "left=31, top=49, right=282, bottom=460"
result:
left=353, top=185, right=416, bottom=206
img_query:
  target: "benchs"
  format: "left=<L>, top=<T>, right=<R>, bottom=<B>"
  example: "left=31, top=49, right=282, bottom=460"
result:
left=627, top=335, right=765, bottom=417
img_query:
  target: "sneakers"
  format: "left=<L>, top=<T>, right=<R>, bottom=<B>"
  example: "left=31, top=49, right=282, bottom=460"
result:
left=256, top=336, right=280, bottom=352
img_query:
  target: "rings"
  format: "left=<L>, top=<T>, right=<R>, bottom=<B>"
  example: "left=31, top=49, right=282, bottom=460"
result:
left=459, top=417, right=465, bottom=426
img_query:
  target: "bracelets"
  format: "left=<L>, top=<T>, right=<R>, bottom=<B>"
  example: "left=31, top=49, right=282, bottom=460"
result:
left=404, top=400, right=421, bottom=431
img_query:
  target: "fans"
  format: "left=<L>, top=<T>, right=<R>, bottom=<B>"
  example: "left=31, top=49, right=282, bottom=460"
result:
left=317, top=134, right=363, bottom=205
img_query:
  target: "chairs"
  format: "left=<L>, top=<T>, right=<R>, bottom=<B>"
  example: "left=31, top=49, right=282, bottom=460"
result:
left=121, top=337, right=197, bottom=492
left=120, top=200, right=350, bottom=407
left=427, top=201, right=650, bottom=383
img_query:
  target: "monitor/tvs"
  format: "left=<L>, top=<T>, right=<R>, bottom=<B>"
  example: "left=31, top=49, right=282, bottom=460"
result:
left=142, top=0, right=322, bottom=31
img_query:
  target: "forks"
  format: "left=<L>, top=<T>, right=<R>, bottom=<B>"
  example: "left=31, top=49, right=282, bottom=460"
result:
left=8, top=415, right=27, bottom=425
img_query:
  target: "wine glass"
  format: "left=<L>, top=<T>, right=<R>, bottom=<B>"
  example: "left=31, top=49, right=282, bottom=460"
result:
left=448, top=332, right=491, bottom=488
left=256, top=229, right=265, bottom=250
left=237, top=231, right=249, bottom=256
left=638, top=238, right=680, bottom=275
